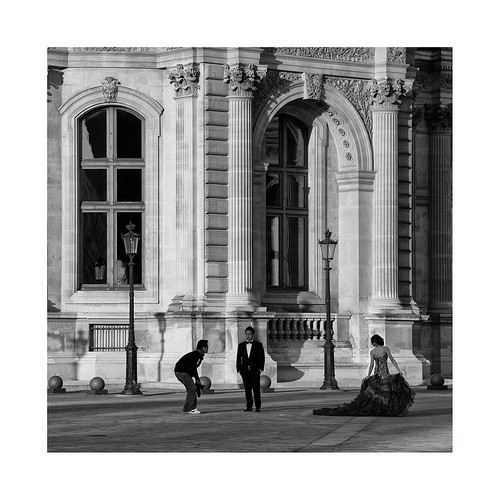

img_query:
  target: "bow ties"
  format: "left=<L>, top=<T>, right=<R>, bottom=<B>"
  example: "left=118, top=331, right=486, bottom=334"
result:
left=246, top=341, right=253, bottom=344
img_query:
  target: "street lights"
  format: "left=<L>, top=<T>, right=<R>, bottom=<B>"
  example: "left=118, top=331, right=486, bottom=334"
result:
left=121, top=220, right=142, bottom=396
left=316, top=228, right=341, bottom=390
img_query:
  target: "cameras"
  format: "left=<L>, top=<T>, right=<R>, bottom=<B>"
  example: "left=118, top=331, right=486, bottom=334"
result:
left=195, top=383, right=205, bottom=390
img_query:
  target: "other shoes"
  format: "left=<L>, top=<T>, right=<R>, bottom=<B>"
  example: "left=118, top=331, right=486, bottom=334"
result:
left=182, top=408, right=202, bottom=414
left=244, top=406, right=252, bottom=411
left=256, top=409, right=263, bottom=412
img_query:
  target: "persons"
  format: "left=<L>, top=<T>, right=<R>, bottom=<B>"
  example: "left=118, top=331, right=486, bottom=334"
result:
left=360, top=334, right=409, bottom=418
left=236, top=326, right=265, bottom=413
left=174, top=339, right=209, bottom=414
